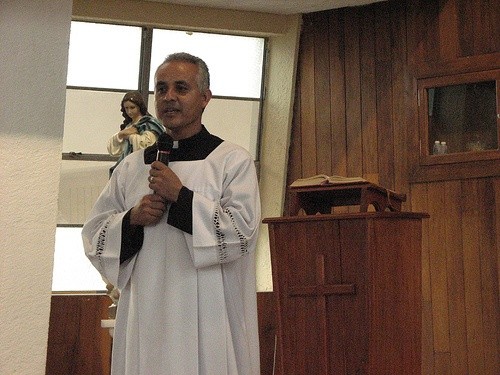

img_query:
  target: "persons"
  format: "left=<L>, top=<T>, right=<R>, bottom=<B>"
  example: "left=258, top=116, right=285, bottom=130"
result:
left=79, top=52, right=262, bottom=375
left=106, top=91, right=166, bottom=179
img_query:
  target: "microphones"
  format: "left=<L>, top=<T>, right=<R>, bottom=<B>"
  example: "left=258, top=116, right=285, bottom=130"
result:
left=154, top=131, right=173, bottom=195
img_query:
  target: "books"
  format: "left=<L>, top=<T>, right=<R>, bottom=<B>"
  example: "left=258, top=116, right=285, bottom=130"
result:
left=289, top=174, right=371, bottom=187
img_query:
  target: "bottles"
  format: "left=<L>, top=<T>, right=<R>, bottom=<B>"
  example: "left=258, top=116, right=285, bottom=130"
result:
left=441, top=142, right=448, bottom=153
left=432, top=141, right=442, bottom=154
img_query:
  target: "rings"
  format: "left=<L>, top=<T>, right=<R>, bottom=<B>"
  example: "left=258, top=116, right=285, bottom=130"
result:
left=150, top=176, right=154, bottom=181
